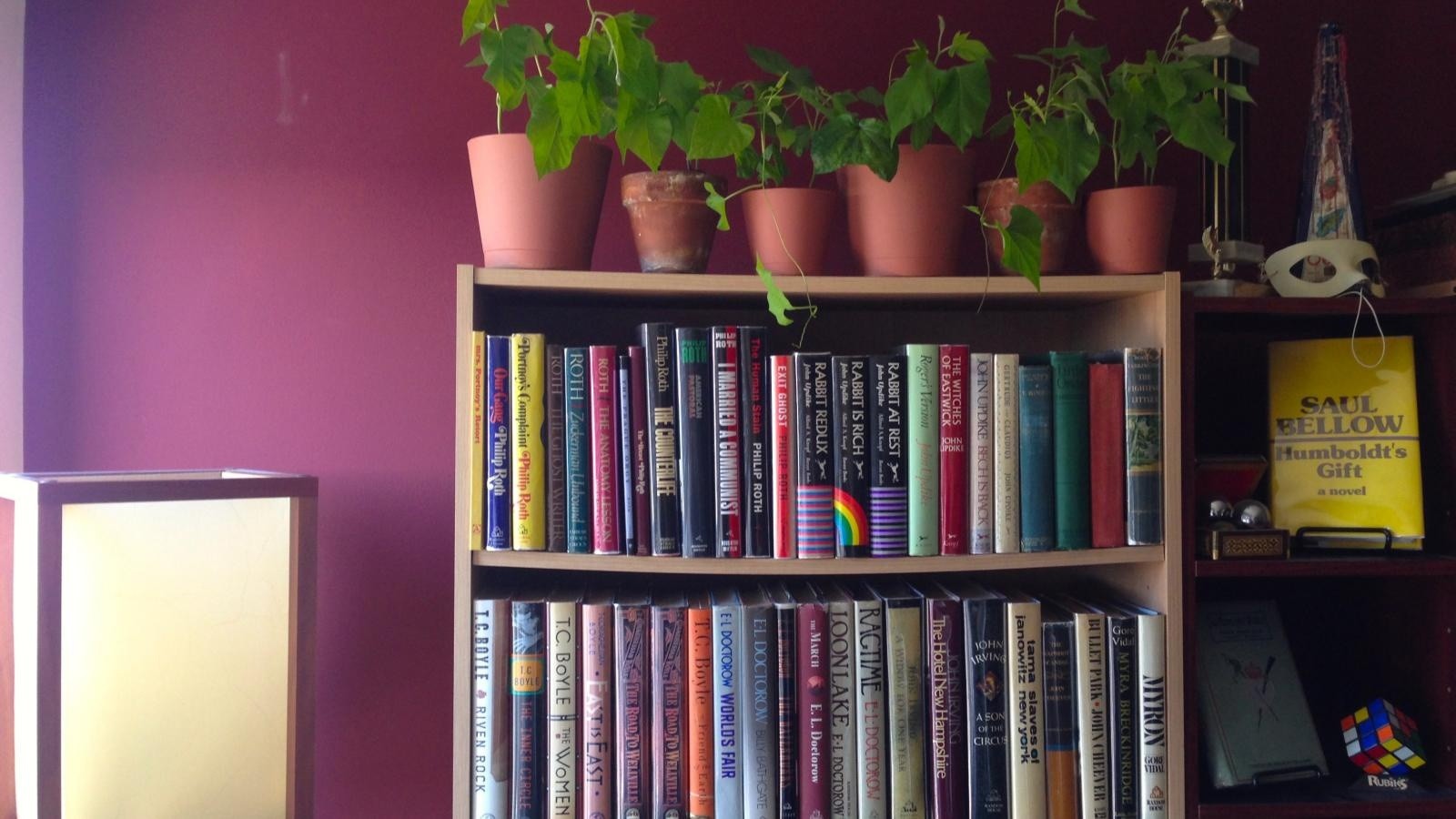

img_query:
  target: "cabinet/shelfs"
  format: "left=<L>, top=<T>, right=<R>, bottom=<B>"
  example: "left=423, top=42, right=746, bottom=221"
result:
left=1179, top=284, right=1456, bottom=819
left=454, top=265, right=1185, bottom=819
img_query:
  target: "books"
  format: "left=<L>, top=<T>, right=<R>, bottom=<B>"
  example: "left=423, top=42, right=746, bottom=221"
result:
left=1266, top=335, right=1425, bottom=551
left=467, top=579, right=1167, bottom=819
left=1194, top=598, right=1332, bottom=800
left=469, top=323, right=1164, bottom=561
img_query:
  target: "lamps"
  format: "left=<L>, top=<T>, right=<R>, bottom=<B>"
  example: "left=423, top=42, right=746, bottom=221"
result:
left=0, top=469, right=318, bottom=819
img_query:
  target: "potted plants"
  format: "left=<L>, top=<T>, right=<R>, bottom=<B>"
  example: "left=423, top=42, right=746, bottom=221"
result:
left=456, top=0, right=1256, bottom=356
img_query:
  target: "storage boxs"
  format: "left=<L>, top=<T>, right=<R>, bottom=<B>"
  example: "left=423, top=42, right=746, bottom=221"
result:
left=1194, top=451, right=1289, bottom=559
